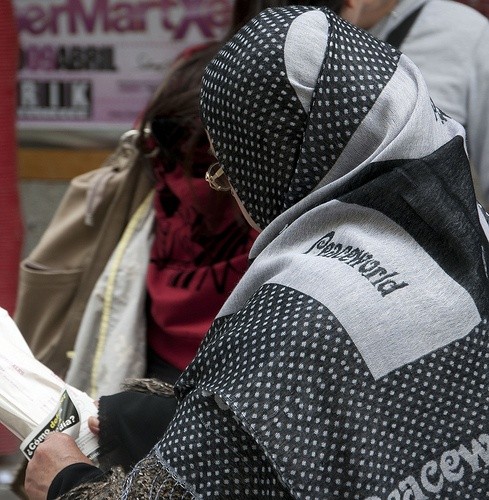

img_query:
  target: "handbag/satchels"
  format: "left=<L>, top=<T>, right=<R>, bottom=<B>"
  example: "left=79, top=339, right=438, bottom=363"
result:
left=10, top=128, right=161, bottom=380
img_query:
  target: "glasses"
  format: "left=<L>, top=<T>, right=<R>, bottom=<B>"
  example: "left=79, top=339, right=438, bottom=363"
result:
left=205, top=162, right=232, bottom=192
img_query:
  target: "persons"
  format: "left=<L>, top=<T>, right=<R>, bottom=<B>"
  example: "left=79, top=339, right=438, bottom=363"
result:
left=354, top=0, right=487, bottom=218
left=22, top=3, right=489, bottom=500
left=143, top=0, right=363, bottom=389
